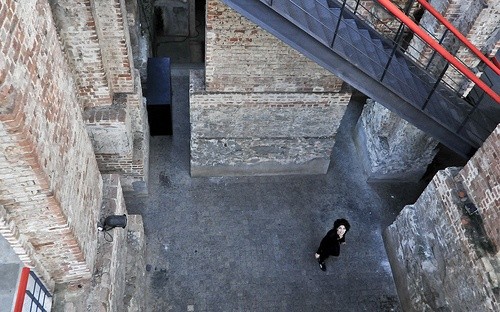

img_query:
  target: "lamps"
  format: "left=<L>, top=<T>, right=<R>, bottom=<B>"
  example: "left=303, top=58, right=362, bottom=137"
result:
left=97, top=213, right=129, bottom=243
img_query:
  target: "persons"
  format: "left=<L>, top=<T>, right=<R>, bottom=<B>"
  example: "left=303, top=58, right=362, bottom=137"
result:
left=315, top=218, right=351, bottom=272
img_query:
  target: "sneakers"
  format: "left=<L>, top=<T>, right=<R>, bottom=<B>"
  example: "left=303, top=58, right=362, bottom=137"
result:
left=318, top=259, right=325, bottom=270
